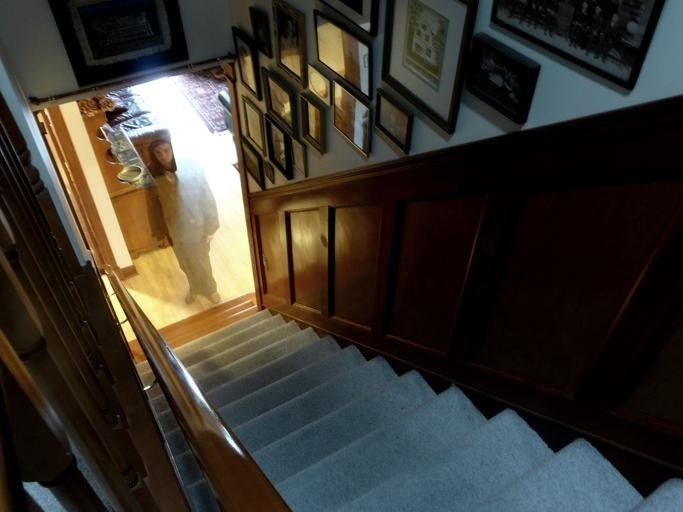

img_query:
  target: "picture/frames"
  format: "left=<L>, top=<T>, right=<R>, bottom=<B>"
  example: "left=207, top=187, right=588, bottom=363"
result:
left=45, top=0, right=190, bottom=91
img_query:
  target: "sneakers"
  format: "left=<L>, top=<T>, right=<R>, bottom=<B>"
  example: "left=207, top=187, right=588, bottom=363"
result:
left=205, top=290, right=220, bottom=304
left=184, top=291, right=197, bottom=305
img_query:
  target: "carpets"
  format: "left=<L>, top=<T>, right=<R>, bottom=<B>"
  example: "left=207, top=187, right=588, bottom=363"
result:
left=172, top=69, right=231, bottom=137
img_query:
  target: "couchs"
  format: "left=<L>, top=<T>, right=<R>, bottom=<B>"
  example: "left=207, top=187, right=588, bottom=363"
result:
left=94, top=86, right=176, bottom=177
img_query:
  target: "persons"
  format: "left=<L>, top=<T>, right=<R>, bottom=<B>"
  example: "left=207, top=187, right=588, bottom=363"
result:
left=506, top=0, right=623, bottom=67
left=142, top=131, right=222, bottom=306
